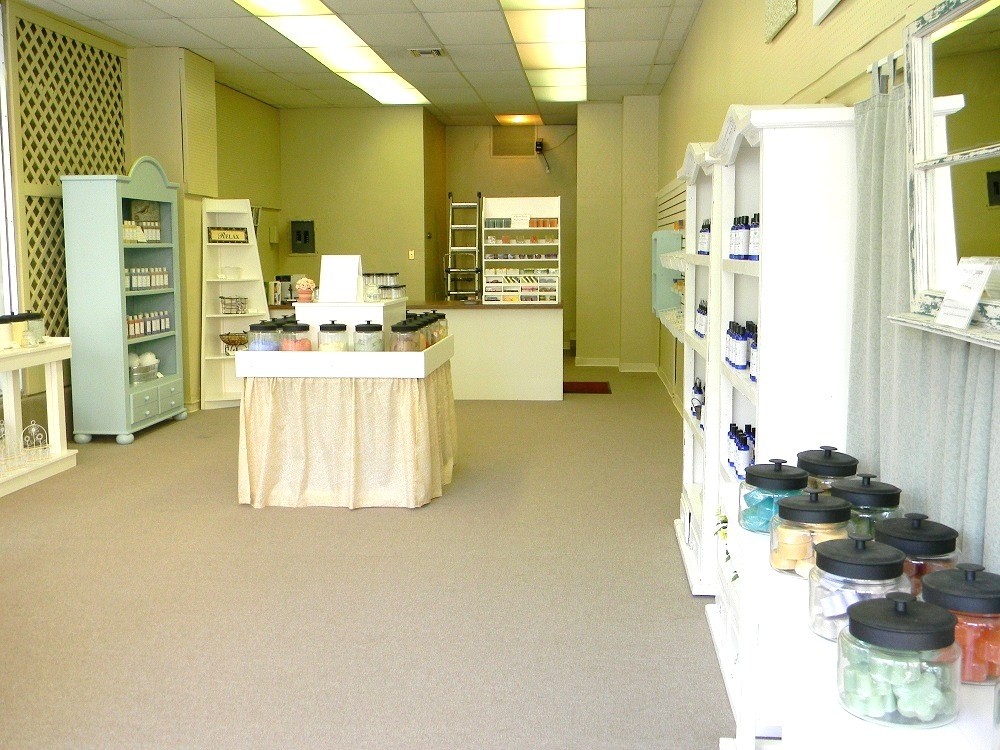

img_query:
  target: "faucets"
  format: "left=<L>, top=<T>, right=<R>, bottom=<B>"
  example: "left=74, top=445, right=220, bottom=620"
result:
left=287, top=284, right=292, bottom=299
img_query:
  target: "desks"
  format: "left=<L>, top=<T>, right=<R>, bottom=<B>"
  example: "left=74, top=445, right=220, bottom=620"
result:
left=0, top=336, right=78, bottom=497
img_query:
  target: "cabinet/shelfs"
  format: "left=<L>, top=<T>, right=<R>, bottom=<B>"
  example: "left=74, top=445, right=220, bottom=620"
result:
left=202, top=197, right=272, bottom=409
left=674, top=104, right=855, bottom=596
left=481, top=195, right=561, bottom=308
left=61, top=156, right=187, bottom=444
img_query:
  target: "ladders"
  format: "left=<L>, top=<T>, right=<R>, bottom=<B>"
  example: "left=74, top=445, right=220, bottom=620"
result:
left=447, top=192, right=481, bottom=301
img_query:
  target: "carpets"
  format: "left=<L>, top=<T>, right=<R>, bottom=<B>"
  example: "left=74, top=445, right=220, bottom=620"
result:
left=564, top=381, right=611, bottom=394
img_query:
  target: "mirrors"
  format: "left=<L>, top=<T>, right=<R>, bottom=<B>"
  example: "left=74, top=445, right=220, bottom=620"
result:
left=921, top=1, right=1000, bottom=303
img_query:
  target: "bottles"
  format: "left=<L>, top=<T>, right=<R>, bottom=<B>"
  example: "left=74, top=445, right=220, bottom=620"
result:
left=0, top=310, right=44, bottom=349
left=249, top=272, right=448, bottom=355
left=484, top=217, right=558, bottom=229
left=689, top=211, right=1000, bottom=731
left=120, top=218, right=171, bottom=339
